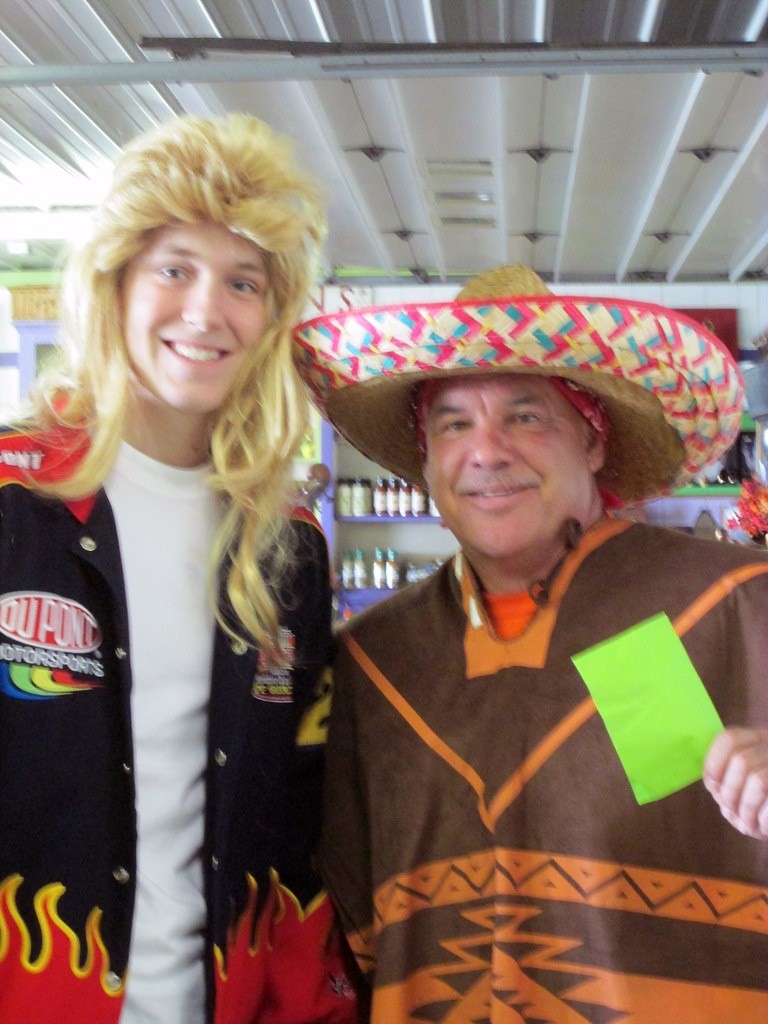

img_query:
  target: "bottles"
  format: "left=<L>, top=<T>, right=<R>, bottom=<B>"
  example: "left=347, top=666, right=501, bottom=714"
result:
left=342, top=547, right=399, bottom=590
left=336, top=476, right=441, bottom=517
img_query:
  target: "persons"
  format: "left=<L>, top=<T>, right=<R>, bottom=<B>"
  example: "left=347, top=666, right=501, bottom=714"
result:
left=314, top=263, right=768, bottom=1024
left=0, top=113, right=333, bottom=1024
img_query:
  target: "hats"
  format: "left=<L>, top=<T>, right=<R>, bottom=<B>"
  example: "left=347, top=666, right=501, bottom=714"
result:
left=289, top=265, right=746, bottom=511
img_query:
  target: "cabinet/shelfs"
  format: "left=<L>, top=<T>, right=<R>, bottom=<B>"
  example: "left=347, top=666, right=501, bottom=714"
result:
left=322, top=415, right=461, bottom=616
